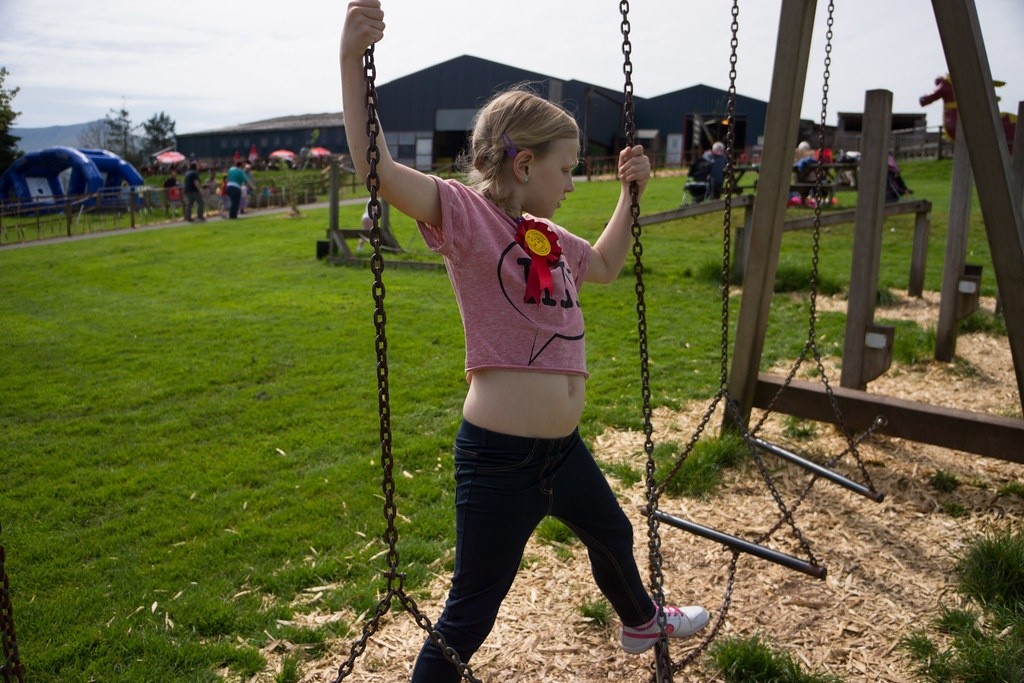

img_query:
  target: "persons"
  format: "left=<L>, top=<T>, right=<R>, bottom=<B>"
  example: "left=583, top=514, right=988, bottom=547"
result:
left=226, top=162, right=258, bottom=219
left=165, top=169, right=276, bottom=217
left=341, top=0, right=711, bottom=683
left=787, top=140, right=913, bottom=208
left=184, top=160, right=206, bottom=221
left=682, top=142, right=727, bottom=204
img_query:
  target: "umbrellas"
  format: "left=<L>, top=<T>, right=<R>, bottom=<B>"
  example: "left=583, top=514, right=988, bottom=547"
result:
left=307, top=147, right=332, bottom=169
left=233, top=151, right=239, bottom=165
left=268, top=149, right=297, bottom=163
left=248, top=144, right=258, bottom=165
left=154, top=147, right=185, bottom=165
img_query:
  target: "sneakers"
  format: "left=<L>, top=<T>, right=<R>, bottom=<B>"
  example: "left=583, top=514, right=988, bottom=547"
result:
left=619, top=599, right=710, bottom=654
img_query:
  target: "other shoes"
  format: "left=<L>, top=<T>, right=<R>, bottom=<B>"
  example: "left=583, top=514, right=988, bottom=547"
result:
left=186, top=217, right=194, bottom=221
left=199, top=217, right=206, bottom=221
